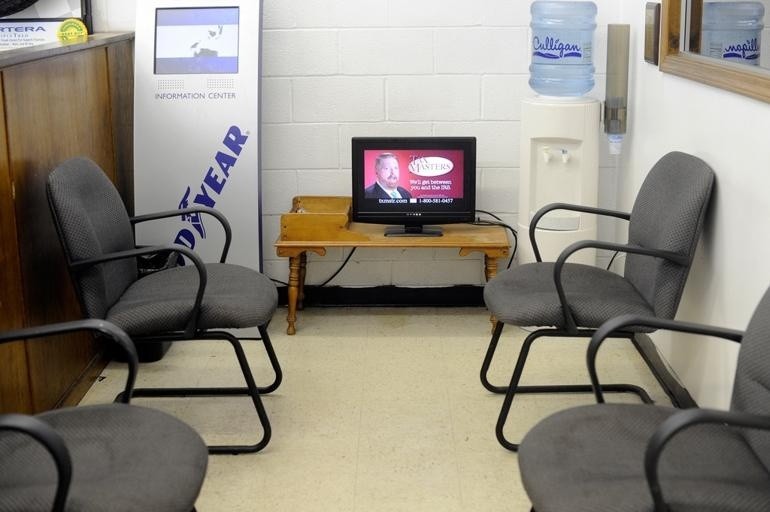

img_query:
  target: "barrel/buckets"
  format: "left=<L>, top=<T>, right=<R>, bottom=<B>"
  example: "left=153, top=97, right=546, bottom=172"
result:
left=700, top=0, right=763, bottom=63
left=529, top=0, right=599, bottom=95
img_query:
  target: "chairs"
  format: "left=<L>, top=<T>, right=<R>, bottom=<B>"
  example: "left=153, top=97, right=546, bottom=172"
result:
left=46, top=154, right=283, bottom=456
left=479, top=152, right=716, bottom=451
left=0, top=319, right=208, bottom=512
left=517, top=287, right=770, bottom=512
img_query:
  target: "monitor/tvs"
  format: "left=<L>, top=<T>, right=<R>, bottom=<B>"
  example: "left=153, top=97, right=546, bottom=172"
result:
left=155, top=7, right=238, bottom=75
left=351, top=137, right=476, bottom=235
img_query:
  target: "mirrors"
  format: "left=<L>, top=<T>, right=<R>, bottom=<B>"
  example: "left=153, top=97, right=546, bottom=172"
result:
left=658, top=1, right=770, bottom=105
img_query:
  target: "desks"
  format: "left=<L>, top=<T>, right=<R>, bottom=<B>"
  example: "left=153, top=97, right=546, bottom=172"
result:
left=275, top=196, right=512, bottom=335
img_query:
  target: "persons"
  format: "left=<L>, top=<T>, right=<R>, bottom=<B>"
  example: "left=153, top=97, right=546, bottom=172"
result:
left=364, top=153, right=412, bottom=198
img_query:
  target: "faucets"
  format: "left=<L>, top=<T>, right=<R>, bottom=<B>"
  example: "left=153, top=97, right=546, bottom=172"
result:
left=540, top=147, right=551, bottom=164
left=559, top=146, right=570, bottom=165
